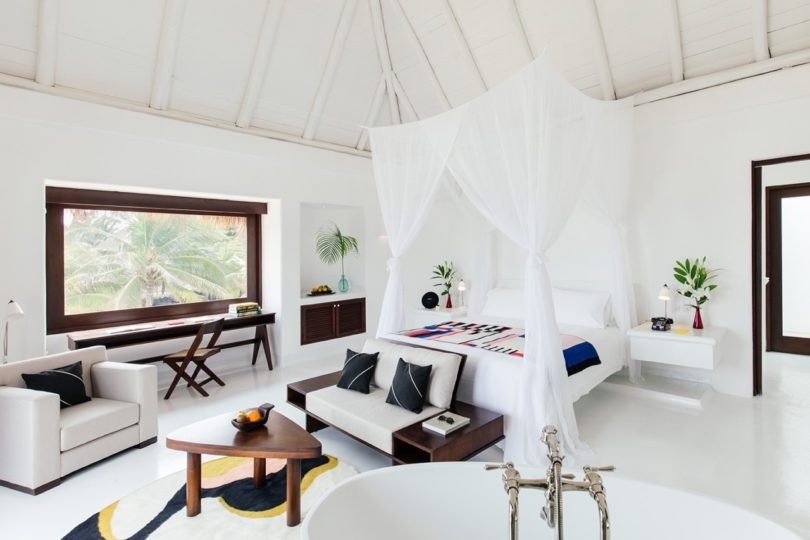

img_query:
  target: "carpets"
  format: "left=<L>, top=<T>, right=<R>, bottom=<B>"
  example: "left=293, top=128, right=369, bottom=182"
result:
left=61, top=454, right=357, bottom=540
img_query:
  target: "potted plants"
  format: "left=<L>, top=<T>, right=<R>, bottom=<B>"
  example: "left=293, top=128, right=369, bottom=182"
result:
left=431, top=261, right=454, bottom=308
left=673, top=257, right=721, bottom=328
left=315, top=223, right=360, bottom=293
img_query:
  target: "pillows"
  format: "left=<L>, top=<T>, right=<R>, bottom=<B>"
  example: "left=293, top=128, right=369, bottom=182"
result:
left=337, top=348, right=380, bottom=394
left=386, top=357, right=433, bottom=414
left=554, top=288, right=611, bottom=328
left=480, top=288, right=525, bottom=319
left=21, top=360, right=92, bottom=410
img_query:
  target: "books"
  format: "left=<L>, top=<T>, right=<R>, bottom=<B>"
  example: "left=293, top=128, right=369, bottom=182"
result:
left=229, top=302, right=259, bottom=317
left=422, top=412, right=471, bottom=435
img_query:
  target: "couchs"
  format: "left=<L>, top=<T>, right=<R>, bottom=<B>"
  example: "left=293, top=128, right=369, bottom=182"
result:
left=0, top=345, right=158, bottom=495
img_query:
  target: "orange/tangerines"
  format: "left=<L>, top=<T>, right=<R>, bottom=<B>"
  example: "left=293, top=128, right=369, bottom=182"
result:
left=246, top=410, right=261, bottom=422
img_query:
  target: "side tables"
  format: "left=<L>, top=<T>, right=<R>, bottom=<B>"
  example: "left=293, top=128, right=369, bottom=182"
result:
left=628, top=321, right=725, bottom=370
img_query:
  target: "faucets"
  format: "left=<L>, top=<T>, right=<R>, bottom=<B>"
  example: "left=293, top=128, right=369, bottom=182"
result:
left=536, top=425, right=566, bottom=529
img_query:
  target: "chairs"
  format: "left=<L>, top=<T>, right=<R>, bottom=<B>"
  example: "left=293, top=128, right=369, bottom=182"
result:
left=163, top=317, right=225, bottom=400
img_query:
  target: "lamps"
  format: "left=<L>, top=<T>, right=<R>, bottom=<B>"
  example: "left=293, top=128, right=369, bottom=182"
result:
left=457, top=279, right=466, bottom=310
left=658, top=284, right=671, bottom=318
left=2, top=300, right=24, bottom=363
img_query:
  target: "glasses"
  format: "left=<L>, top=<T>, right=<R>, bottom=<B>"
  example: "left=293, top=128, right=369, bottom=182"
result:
left=438, top=416, right=455, bottom=424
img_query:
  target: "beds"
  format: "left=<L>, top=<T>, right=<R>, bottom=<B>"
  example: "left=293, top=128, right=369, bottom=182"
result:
left=363, top=285, right=630, bottom=416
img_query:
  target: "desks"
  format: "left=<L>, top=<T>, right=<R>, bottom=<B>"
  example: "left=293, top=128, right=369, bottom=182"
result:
left=166, top=406, right=322, bottom=527
left=67, top=310, right=275, bottom=371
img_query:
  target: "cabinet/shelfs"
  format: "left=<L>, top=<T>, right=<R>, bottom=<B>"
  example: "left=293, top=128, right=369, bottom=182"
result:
left=301, top=293, right=367, bottom=345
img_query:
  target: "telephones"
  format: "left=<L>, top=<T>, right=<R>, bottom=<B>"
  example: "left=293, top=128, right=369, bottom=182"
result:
left=651, top=316, right=673, bottom=331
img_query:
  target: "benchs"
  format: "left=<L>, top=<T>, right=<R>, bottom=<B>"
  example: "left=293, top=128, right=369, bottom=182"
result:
left=286, top=337, right=506, bottom=466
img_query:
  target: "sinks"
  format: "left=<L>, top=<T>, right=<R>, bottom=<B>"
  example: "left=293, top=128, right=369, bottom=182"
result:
left=301, top=457, right=801, bottom=540
